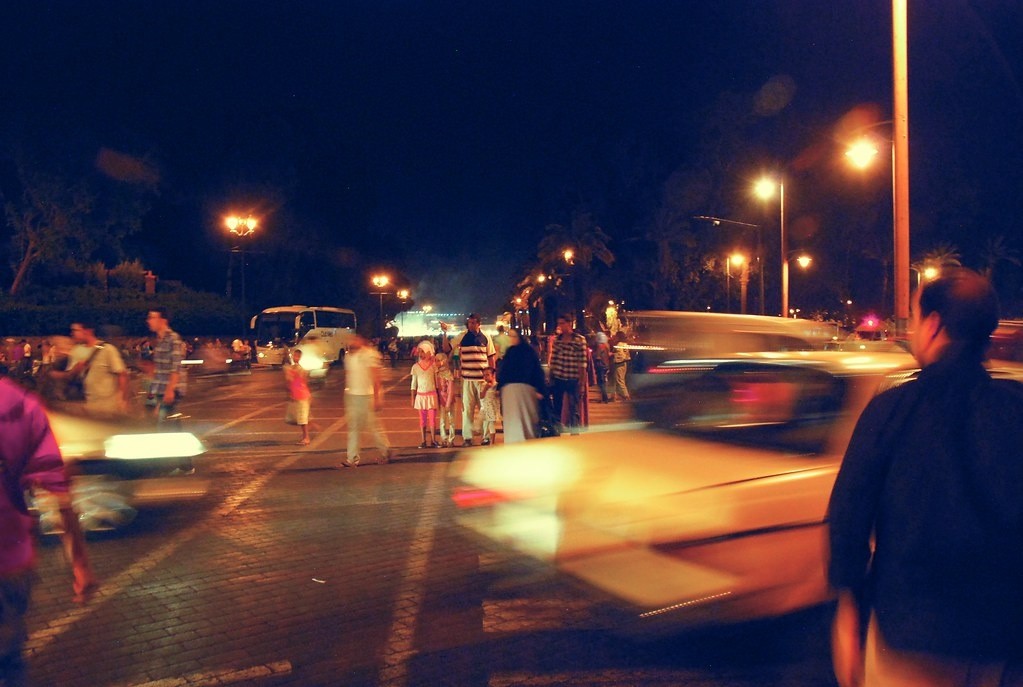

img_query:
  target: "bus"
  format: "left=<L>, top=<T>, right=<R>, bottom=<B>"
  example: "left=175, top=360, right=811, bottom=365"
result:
left=249, top=304, right=357, bottom=369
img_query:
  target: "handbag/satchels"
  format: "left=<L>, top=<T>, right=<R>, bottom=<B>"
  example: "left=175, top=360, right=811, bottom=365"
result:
left=613, top=349, right=631, bottom=364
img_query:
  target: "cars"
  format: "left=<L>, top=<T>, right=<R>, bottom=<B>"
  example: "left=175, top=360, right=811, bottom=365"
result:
left=447, top=347, right=1022, bottom=644
left=17, top=404, right=209, bottom=543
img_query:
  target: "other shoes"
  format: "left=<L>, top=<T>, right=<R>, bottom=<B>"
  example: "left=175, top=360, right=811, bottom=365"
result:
left=418, top=442, right=428, bottom=448
left=621, top=396, right=631, bottom=402
left=375, top=455, right=394, bottom=465
left=430, top=441, right=442, bottom=448
left=335, top=458, right=361, bottom=469
left=609, top=397, right=618, bottom=402
left=463, top=439, right=473, bottom=447
left=481, top=438, right=489, bottom=445
left=448, top=441, right=456, bottom=448
left=599, top=400, right=609, bottom=404
left=440, top=441, right=447, bottom=448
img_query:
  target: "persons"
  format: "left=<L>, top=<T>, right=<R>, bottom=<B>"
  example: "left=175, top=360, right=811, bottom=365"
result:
left=823, top=267, right=1023, bottom=687
left=0, top=307, right=629, bottom=472
left=0, top=373, right=97, bottom=687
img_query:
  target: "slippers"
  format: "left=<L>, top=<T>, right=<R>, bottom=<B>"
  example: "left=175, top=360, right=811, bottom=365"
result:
left=296, top=439, right=310, bottom=446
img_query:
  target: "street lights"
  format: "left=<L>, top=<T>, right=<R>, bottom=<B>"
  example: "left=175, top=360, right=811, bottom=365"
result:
left=752, top=173, right=790, bottom=321
left=225, top=213, right=257, bottom=339
left=423, top=305, right=432, bottom=332
left=726, top=253, right=744, bottom=314
left=369, top=275, right=394, bottom=356
left=844, top=119, right=912, bottom=341
left=395, top=289, right=410, bottom=362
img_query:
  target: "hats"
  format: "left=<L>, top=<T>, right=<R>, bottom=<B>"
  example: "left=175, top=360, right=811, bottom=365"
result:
left=418, top=342, right=434, bottom=356
